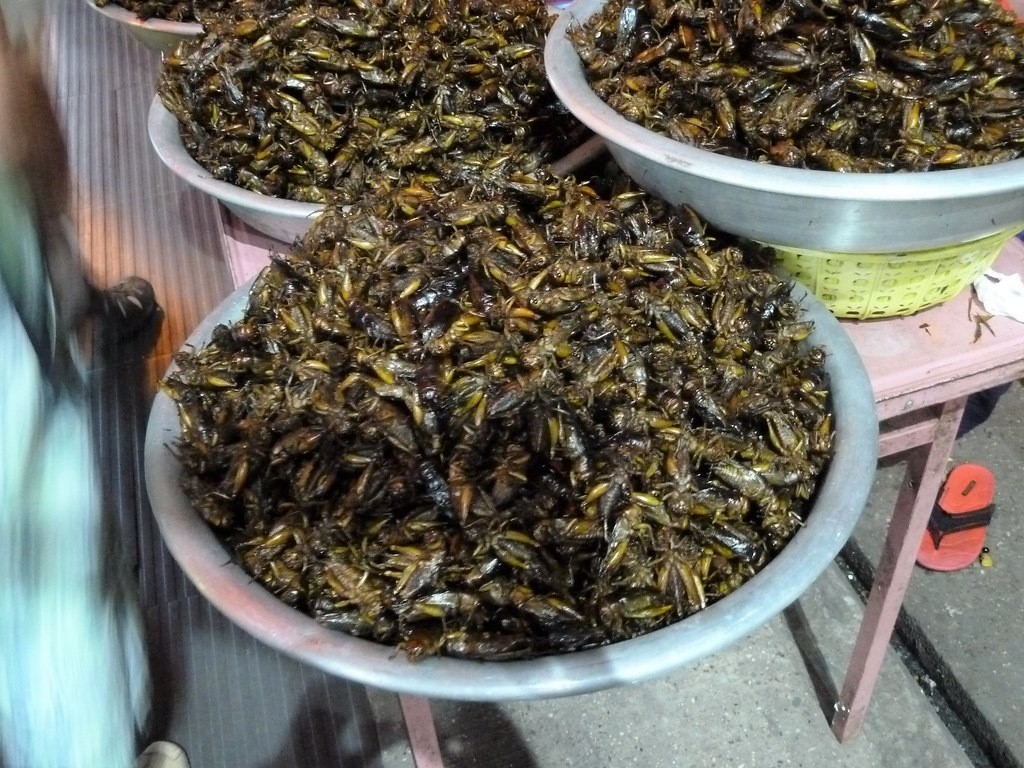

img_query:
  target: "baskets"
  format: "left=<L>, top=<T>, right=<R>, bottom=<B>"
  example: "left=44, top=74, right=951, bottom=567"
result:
left=748, top=224, right=1024, bottom=320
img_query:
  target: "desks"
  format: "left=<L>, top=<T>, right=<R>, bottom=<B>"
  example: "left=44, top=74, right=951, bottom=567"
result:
left=210, top=198, right=1024, bottom=767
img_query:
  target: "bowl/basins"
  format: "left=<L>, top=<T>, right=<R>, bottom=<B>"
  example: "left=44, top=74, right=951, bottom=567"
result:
left=148, top=93, right=606, bottom=243
left=87, top=0, right=570, bottom=53
left=145, top=266, right=879, bottom=703
left=545, top=0, right=1024, bottom=254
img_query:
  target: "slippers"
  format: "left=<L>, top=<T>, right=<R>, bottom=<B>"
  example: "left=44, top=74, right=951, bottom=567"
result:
left=916, top=464, right=996, bottom=571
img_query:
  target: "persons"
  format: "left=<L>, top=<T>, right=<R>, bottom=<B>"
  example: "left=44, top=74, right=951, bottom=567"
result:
left=0, top=0, right=189, bottom=768
